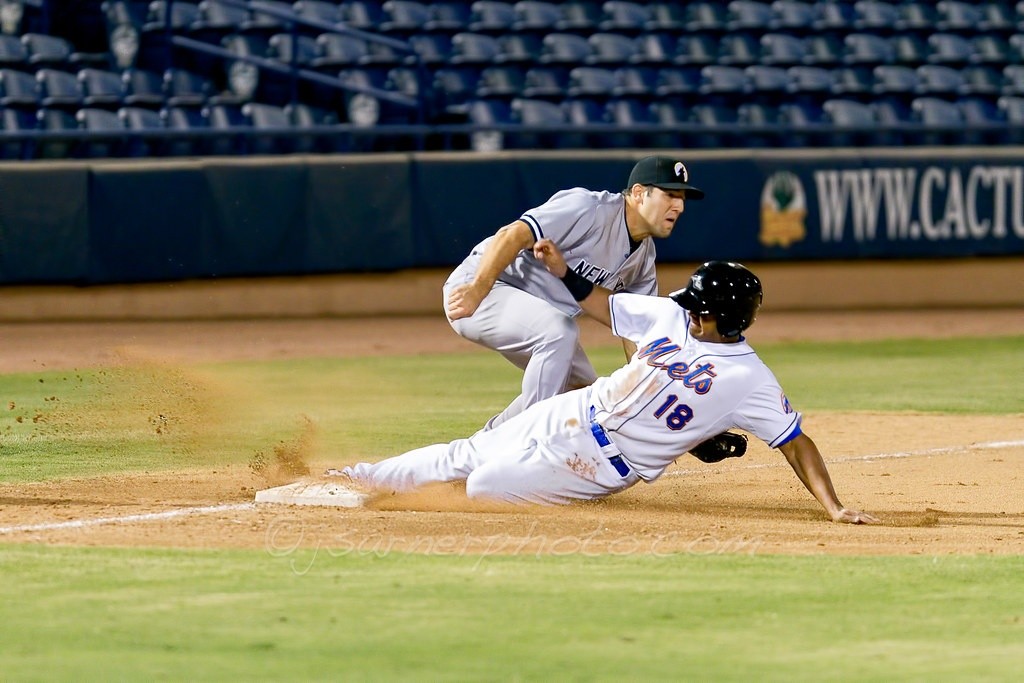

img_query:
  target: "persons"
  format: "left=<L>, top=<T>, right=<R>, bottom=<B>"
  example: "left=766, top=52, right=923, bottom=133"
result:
left=324, top=238, right=880, bottom=525
left=444, top=155, right=746, bottom=463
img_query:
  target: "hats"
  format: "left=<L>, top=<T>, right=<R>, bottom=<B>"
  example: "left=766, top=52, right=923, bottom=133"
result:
left=627, top=155, right=699, bottom=192
left=670, top=261, right=763, bottom=337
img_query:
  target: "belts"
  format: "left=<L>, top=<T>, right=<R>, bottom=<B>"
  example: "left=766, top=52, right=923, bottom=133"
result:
left=588, top=391, right=630, bottom=479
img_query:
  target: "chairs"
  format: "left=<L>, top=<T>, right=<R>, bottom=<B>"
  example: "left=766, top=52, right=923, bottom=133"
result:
left=1, top=1, right=1024, bottom=132
